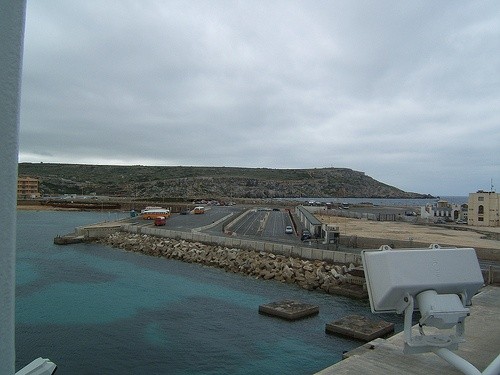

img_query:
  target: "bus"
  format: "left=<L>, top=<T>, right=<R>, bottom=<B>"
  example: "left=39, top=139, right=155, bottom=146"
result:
left=142, top=209, right=169, bottom=220
left=165, top=210, right=170, bottom=217
left=194, top=207, right=205, bottom=214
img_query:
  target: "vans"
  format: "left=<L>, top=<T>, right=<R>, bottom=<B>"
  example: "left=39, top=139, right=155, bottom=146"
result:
left=155, top=216, right=167, bottom=226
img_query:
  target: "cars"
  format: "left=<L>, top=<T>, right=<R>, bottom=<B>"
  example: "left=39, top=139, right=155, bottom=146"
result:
left=299, top=230, right=312, bottom=240
left=285, top=225, right=292, bottom=233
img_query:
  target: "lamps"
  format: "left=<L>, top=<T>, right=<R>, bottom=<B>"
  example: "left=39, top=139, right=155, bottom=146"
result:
left=361, top=242, right=483, bottom=348
